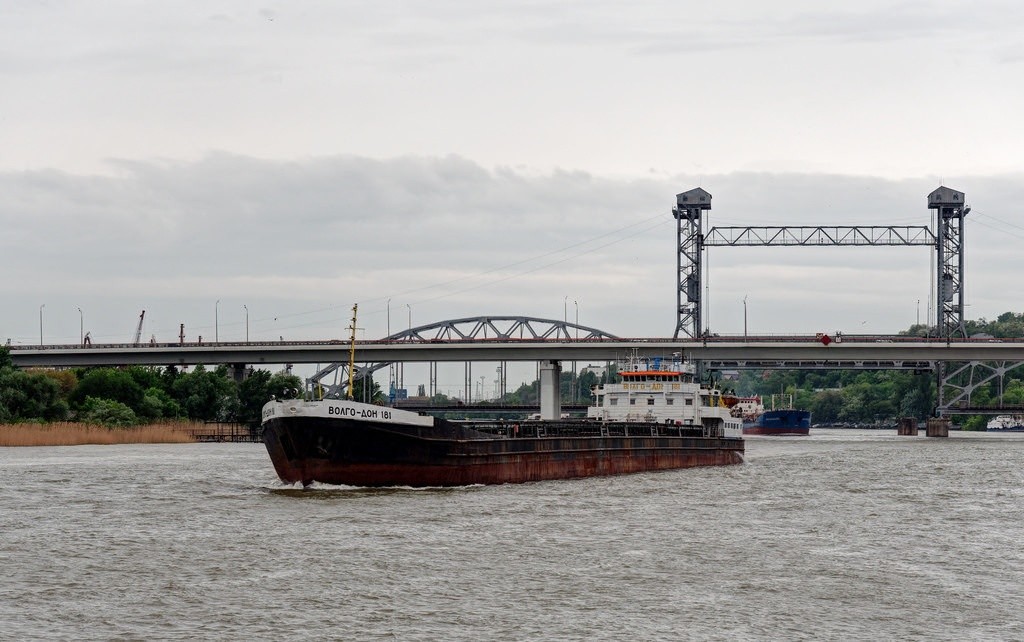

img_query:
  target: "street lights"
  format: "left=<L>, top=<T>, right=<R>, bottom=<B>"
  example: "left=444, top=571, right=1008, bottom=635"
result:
left=387, top=299, right=393, bottom=344
left=243, top=304, right=249, bottom=346
left=742, top=294, right=749, bottom=342
left=574, top=301, right=579, bottom=343
left=78, top=306, right=85, bottom=350
left=564, top=295, right=569, bottom=321
left=406, top=304, right=412, bottom=340
left=39, top=303, right=46, bottom=347
left=215, top=298, right=222, bottom=347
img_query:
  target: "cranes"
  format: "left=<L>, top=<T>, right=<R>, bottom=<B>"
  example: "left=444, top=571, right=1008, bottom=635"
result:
left=133, top=310, right=146, bottom=343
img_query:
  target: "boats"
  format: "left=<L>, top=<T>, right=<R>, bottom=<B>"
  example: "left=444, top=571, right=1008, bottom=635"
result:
left=743, top=393, right=814, bottom=436
left=257, top=303, right=749, bottom=491
